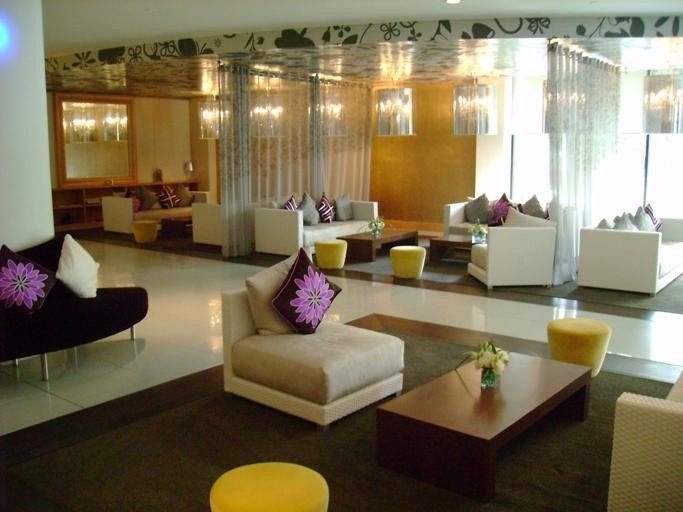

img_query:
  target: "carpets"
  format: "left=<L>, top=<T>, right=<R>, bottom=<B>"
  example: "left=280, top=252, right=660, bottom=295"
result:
left=0, top=314, right=673, bottom=512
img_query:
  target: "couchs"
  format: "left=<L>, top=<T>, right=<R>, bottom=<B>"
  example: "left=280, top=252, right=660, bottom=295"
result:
left=192, top=201, right=257, bottom=247
left=255, top=199, right=378, bottom=256
left=101, top=190, right=209, bottom=234
left=443, top=201, right=574, bottom=258
left=0, top=238, right=148, bottom=382
left=577, top=217, right=683, bottom=298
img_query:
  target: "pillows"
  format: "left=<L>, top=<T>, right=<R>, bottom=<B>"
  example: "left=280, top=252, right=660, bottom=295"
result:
left=269, top=246, right=343, bottom=336
left=124, top=183, right=193, bottom=212
left=464, top=192, right=557, bottom=227
left=270, top=191, right=354, bottom=225
left=54, top=234, right=100, bottom=299
left=245, top=241, right=313, bottom=335
left=1, top=244, right=57, bottom=317
left=596, top=203, right=662, bottom=232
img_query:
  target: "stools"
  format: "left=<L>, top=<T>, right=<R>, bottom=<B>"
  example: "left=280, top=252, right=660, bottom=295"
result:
left=390, top=245, right=426, bottom=280
left=208, top=461, right=330, bottom=512
left=547, top=319, right=612, bottom=377
left=132, top=220, right=158, bottom=244
left=315, top=239, right=348, bottom=270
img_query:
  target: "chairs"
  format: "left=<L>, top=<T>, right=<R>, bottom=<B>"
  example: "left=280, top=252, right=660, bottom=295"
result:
left=220, top=285, right=406, bottom=430
left=467, top=226, right=557, bottom=289
left=606, top=370, right=683, bottom=511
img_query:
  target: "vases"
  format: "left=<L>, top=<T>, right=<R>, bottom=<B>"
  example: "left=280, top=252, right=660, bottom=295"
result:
left=480, top=367, right=498, bottom=390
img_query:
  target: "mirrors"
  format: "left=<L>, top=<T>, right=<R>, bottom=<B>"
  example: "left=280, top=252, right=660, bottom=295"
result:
left=55, top=93, right=138, bottom=188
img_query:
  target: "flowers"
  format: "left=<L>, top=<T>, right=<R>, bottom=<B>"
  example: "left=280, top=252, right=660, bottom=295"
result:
left=463, top=335, right=510, bottom=374
